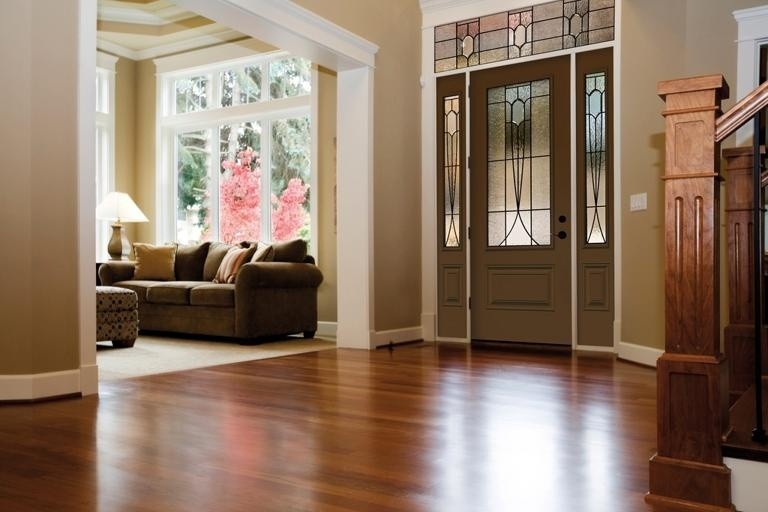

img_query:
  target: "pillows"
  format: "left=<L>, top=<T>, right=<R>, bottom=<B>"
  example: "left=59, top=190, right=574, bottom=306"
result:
left=131, top=238, right=307, bottom=285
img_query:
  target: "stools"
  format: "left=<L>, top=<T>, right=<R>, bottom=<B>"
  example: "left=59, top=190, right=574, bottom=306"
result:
left=98, top=285, right=140, bottom=349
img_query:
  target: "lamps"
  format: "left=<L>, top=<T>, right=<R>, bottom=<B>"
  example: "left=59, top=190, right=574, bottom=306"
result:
left=95, top=192, right=149, bottom=260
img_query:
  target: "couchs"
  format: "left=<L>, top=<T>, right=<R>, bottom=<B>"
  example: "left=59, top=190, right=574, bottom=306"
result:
left=97, top=238, right=324, bottom=346
